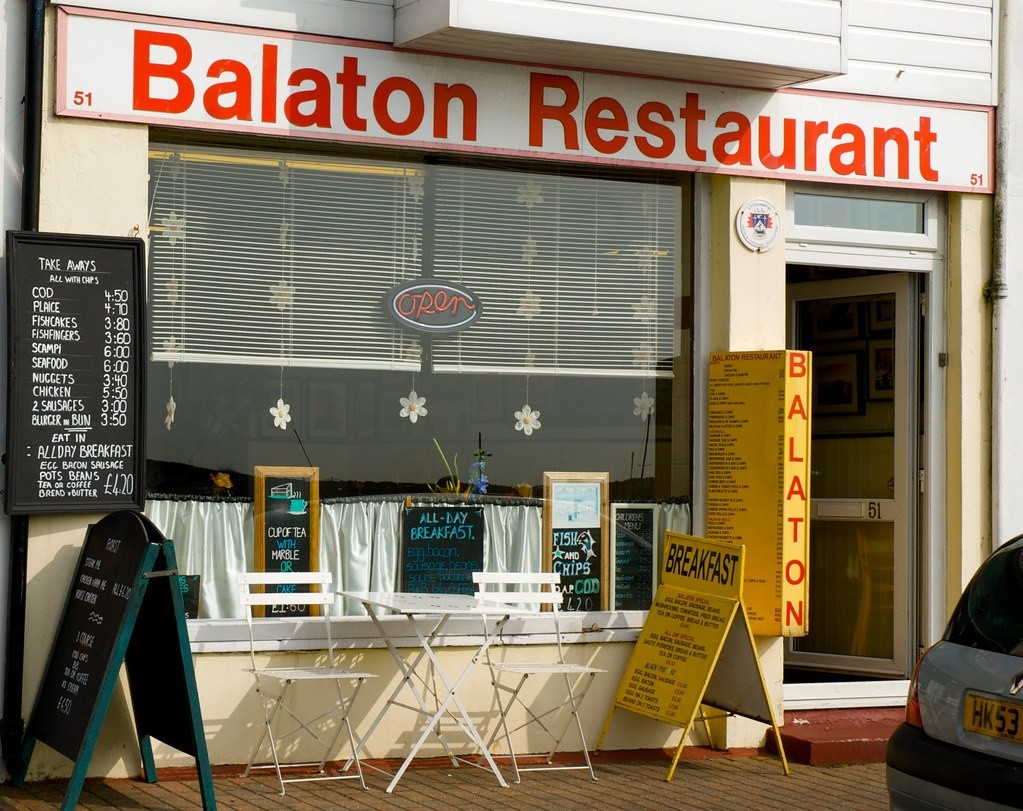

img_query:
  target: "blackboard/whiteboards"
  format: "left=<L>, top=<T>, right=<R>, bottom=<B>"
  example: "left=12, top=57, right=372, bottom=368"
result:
left=27, top=512, right=207, bottom=763
left=541, top=528, right=610, bottom=612
left=5, top=229, right=145, bottom=515
left=611, top=503, right=658, bottom=611
left=403, top=507, right=485, bottom=597
left=179, top=574, right=200, bottom=619
left=255, top=465, right=319, bottom=618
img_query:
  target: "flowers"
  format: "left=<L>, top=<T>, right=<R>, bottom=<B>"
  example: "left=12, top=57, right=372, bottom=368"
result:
left=211, top=472, right=233, bottom=488
left=269, top=162, right=296, bottom=430
left=161, top=150, right=185, bottom=431
left=513, top=177, right=543, bottom=436
left=632, top=185, right=659, bottom=420
left=398, top=169, right=429, bottom=423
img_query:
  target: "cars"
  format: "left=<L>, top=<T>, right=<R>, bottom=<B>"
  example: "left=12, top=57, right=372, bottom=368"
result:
left=884, top=533, right=1023, bottom=811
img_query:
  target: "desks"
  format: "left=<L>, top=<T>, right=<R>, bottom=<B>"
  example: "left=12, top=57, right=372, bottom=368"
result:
left=334, top=588, right=539, bottom=793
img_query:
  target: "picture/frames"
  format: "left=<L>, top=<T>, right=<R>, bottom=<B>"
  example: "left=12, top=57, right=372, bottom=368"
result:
left=541, top=472, right=610, bottom=612
left=255, top=378, right=303, bottom=442
left=254, top=466, right=321, bottom=618
left=472, top=373, right=508, bottom=424
left=306, top=379, right=349, bottom=440
left=371, top=382, right=410, bottom=437
left=800, top=266, right=898, bottom=416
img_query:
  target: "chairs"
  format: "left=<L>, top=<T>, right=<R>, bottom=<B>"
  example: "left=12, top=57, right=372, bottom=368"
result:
left=236, top=570, right=381, bottom=796
left=470, top=571, right=608, bottom=784
